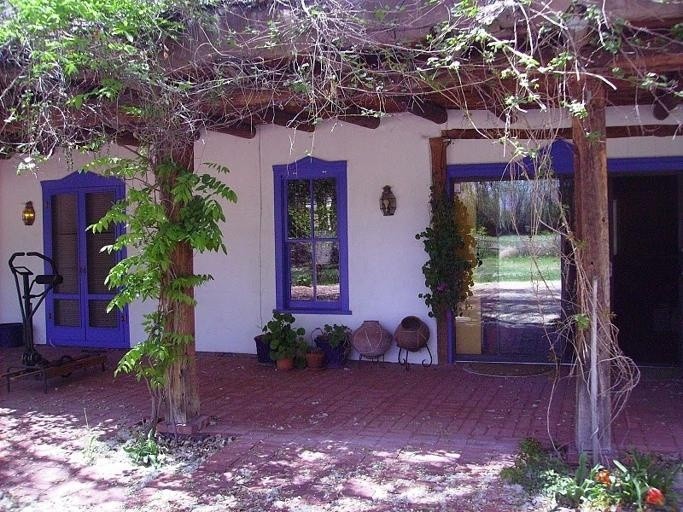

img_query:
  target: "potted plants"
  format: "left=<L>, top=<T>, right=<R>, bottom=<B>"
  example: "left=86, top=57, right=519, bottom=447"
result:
left=254, top=309, right=353, bottom=371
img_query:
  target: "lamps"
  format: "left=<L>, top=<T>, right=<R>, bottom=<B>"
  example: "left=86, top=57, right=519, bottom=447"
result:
left=21, top=201, right=35, bottom=225
left=379, top=184, right=397, bottom=216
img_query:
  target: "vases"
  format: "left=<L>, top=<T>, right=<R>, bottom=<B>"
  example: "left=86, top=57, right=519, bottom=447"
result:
left=350, top=320, right=393, bottom=356
left=394, top=316, right=430, bottom=352
